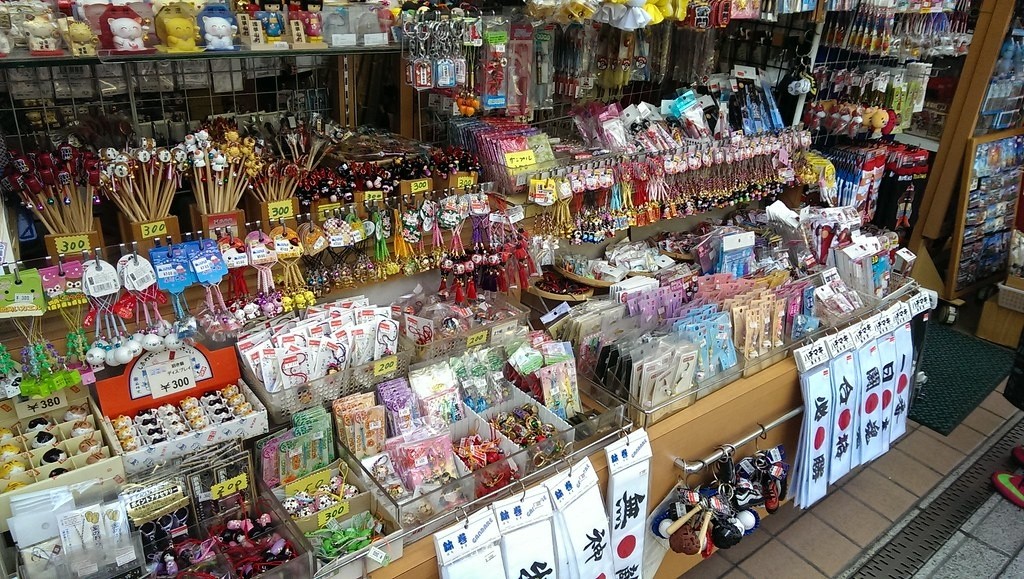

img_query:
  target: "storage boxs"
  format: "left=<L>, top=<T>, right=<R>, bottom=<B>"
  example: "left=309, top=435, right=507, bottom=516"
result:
left=0, top=372, right=626, bottom=579
left=1007, top=274, right=1024, bottom=290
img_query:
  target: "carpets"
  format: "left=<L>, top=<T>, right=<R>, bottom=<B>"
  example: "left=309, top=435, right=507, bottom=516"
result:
left=906, top=321, right=1018, bottom=436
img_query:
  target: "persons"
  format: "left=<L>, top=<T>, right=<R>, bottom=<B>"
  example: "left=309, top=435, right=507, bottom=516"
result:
left=990, top=323, right=1024, bottom=510
left=256, top=0, right=325, bottom=38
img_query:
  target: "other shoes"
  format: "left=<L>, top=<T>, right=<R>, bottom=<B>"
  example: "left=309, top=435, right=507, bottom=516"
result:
left=991, top=470, right=1024, bottom=509
left=1011, top=445, right=1024, bottom=465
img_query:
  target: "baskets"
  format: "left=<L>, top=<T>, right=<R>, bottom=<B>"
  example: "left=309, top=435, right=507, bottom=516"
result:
left=997, top=280, right=1024, bottom=313
